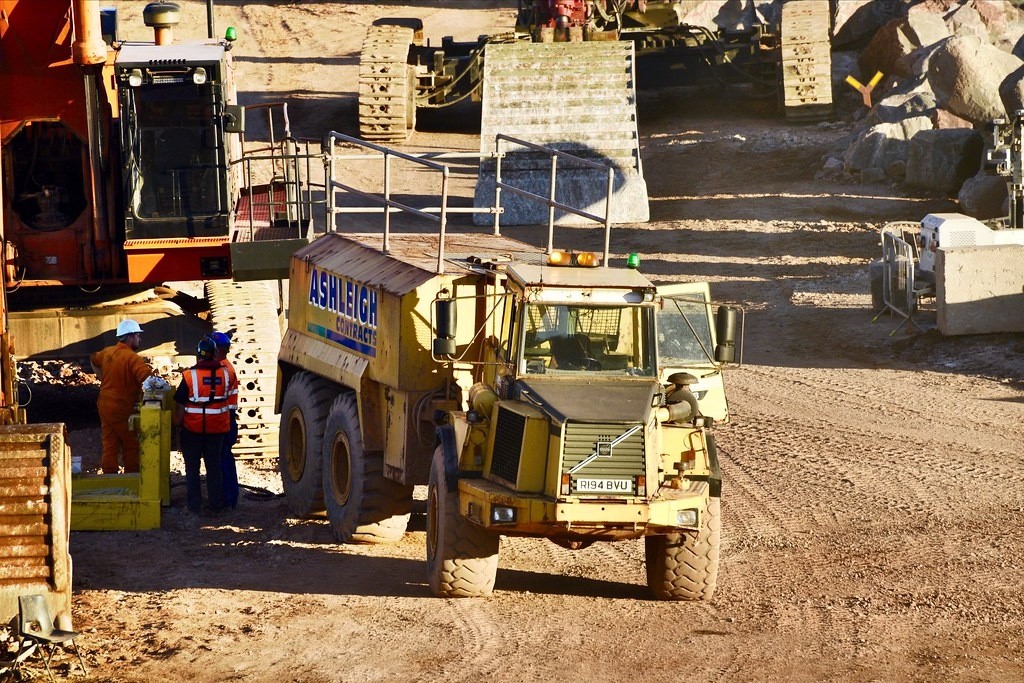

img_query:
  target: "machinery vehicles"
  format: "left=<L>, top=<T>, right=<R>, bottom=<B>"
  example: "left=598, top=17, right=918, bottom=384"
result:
left=354, top=0, right=837, bottom=145
left=0, top=0, right=317, bottom=631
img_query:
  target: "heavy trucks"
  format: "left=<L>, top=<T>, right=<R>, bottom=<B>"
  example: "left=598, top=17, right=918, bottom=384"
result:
left=271, top=128, right=750, bottom=600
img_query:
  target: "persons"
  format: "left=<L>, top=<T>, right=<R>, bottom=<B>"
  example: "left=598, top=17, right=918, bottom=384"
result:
left=174, top=332, right=241, bottom=514
left=90, top=319, right=164, bottom=473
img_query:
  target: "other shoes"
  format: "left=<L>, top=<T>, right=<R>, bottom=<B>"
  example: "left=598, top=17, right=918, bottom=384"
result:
left=182, top=505, right=199, bottom=515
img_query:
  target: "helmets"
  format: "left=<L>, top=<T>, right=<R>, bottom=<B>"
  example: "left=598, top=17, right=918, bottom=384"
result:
left=207, top=332, right=231, bottom=349
left=116, top=320, right=144, bottom=336
left=196, top=337, right=217, bottom=361
